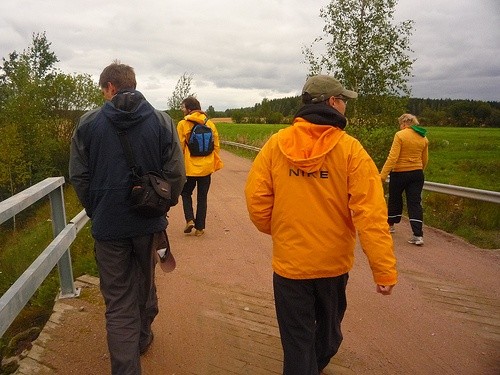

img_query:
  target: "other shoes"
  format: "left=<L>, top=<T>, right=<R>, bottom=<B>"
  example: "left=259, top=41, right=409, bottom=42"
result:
left=408, top=235, right=425, bottom=245
left=183, top=220, right=204, bottom=237
left=389, top=225, right=396, bottom=232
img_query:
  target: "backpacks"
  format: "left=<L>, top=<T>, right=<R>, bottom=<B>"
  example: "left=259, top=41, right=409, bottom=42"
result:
left=183, top=117, right=215, bottom=158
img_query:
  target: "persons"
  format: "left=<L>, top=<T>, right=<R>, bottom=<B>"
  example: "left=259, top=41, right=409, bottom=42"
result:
left=69, top=60, right=186, bottom=375
left=244, top=76, right=397, bottom=375
left=380, top=114, right=429, bottom=245
left=177, top=97, right=224, bottom=237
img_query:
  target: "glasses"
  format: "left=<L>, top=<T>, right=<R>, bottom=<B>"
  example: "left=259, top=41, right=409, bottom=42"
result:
left=325, top=95, right=350, bottom=104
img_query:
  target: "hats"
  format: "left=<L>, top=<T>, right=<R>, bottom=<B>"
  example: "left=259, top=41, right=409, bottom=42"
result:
left=302, top=74, right=358, bottom=104
left=153, top=230, right=176, bottom=273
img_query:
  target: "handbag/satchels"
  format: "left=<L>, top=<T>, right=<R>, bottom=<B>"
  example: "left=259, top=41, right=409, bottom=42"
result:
left=130, top=175, right=173, bottom=216
